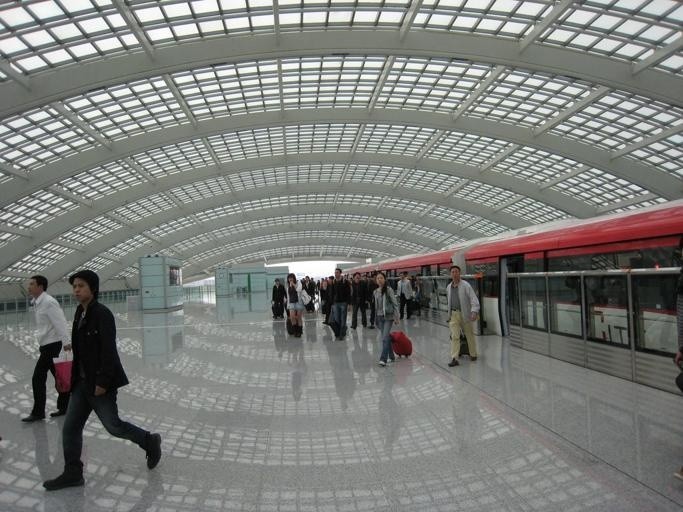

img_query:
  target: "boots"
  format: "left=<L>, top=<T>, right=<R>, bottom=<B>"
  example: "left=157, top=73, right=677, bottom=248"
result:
left=40, top=468, right=86, bottom=491
left=291, top=324, right=297, bottom=336
left=295, top=325, right=302, bottom=337
left=144, top=432, right=162, bottom=470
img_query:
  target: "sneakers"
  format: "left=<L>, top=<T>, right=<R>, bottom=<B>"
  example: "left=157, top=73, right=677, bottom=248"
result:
left=447, top=359, right=458, bottom=367
left=385, top=359, right=396, bottom=363
left=470, top=357, right=477, bottom=361
left=319, top=321, right=375, bottom=339
left=377, top=360, right=386, bottom=366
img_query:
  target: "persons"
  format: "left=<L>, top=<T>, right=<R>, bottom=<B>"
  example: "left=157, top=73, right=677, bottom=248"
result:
left=446, top=266, right=481, bottom=367
left=22, top=275, right=72, bottom=422
left=673, top=266, right=683, bottom=482
left=272, top=267, right=429, bottom=366
left=43, top=270, right=162, bottom=491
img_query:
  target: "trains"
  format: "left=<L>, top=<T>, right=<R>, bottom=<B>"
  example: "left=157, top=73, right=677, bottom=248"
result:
left=337, top=195, right=682, bottom=399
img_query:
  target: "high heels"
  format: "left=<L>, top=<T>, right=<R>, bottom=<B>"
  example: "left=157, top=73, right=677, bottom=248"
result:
left=49, top=408, right=65, bottom=417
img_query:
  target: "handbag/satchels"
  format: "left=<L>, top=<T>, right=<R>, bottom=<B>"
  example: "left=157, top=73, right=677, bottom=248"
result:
left=299, top=289, right=313, bottom=306
left=51, top=343, right=76, bottom=395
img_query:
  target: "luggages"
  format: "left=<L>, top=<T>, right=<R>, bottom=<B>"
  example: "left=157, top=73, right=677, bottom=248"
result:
left=389, top=331, right=413, bottom=359
left=271, top=299, right=285, bottom=319
left=446, top=319, right=470, bottom=358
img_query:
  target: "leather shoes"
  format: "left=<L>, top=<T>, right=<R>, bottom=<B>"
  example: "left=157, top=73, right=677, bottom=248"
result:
left=20, top=412, right=44, bottom=422
